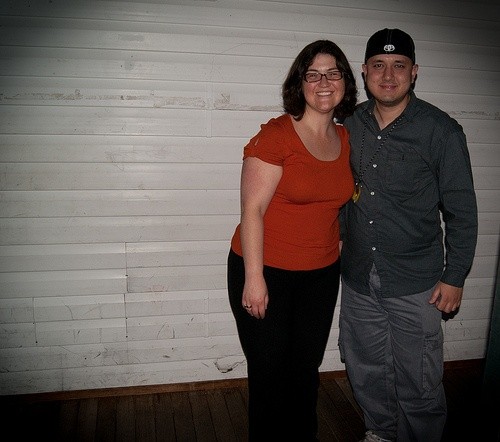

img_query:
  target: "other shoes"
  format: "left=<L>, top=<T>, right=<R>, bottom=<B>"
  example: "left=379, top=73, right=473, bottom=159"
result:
left=360, top=430, right=392, bottom=442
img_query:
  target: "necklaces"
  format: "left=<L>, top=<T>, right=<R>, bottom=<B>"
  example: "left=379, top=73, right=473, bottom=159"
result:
left=351, top=111, right=410, bottom=200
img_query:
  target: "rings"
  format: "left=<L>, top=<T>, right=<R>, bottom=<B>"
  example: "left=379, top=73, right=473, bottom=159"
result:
left=246, top=305, right=252, bottom=308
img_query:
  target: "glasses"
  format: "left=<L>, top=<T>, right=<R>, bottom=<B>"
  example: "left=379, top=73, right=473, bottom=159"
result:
left=303, top=71, right=344, bottom=83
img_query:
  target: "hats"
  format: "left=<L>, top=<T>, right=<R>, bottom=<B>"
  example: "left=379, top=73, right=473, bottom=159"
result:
left=364, top=28, right=415, bottom=65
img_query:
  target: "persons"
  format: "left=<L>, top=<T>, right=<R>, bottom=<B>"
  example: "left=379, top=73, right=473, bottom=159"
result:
left=226, top=40, right=356, bottom=442
left=338, top=28, right=479, bottom=442
